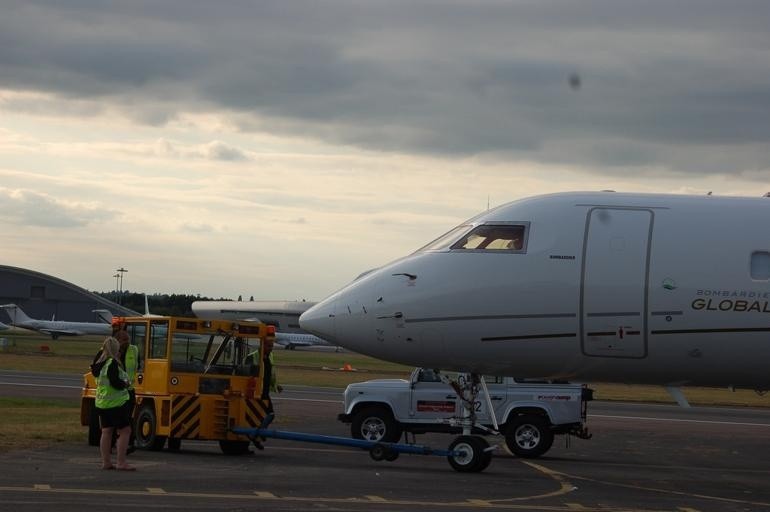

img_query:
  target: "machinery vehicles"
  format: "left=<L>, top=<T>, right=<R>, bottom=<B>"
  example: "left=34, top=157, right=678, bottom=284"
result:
left=80, top=315, right=275, bottom=457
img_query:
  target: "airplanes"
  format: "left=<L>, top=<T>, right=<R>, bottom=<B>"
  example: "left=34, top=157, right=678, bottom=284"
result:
left=295, top=186, right=768, bottom=472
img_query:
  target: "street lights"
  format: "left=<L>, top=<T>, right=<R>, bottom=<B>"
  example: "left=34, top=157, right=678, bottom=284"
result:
left=114, top=274, right=120, bottom=303
left=117, top=268, right=128, bottom=304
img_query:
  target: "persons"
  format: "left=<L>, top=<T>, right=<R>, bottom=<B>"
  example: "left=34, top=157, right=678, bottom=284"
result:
left=242, top=334, right=284, bottom=457
left=92, top=329, right=140, bottom=455
left=90, top=337, right=137, bottom=471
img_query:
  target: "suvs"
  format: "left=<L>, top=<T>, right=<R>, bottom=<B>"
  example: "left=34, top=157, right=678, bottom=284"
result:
left=339, top=367, right=592, bottom=458
